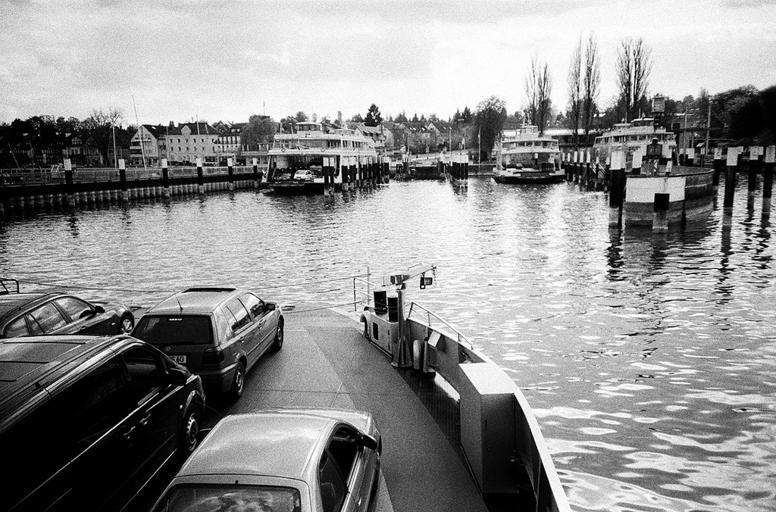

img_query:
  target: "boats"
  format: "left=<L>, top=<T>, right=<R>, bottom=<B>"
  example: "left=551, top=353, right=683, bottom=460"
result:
left=215, top=258, right=574, bottom=511
left=259, top=121, right=390, bottom=200
left=491, top=124, right=567, bottom=184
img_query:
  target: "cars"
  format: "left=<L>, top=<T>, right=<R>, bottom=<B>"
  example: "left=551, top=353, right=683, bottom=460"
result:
left=1, top=291, right=137, bottom=339
left=149, top=406, right=384, bottom=511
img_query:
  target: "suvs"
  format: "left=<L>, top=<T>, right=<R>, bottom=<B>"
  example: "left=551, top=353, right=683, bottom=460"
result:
left=129, top=284, right=285, bottom=399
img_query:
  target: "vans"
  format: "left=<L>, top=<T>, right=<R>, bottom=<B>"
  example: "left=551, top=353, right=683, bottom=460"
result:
left=1, top=334, right=211, bottom=512
